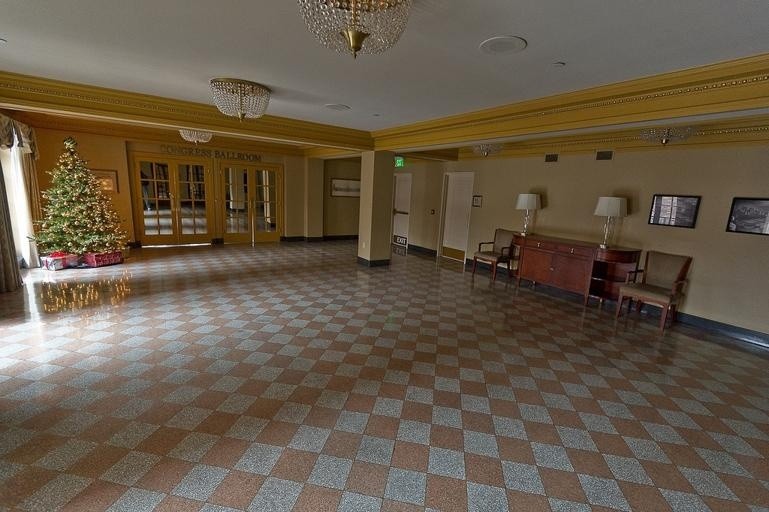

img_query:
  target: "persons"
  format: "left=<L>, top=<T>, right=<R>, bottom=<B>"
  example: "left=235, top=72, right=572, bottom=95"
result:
left=141, top=171, right=152, bottom=211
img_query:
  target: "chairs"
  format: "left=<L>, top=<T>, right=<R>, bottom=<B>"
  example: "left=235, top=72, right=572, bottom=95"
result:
left=616, top=251, right=692, bottom=335
left=471, top=228, right=518, bottom=282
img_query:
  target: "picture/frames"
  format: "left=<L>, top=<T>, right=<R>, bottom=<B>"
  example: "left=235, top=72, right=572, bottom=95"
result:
left=330, top=178, right=360, bottom=198
left=648, top=194, right=769, bottom=235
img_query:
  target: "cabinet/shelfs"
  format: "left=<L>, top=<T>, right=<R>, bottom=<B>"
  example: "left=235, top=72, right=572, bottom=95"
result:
left=513, top=235, right=641, bottom=308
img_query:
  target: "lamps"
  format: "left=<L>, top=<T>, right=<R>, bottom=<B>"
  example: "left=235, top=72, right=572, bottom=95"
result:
left=178, top=129, right=213, bottom=145
left=209, top=79, right=272, bottom=124
left=592, top=197, right=627, bottom=248
left=296, top=0, right=410, bottom=58
left=516, top=193, right=542, bottom=236
